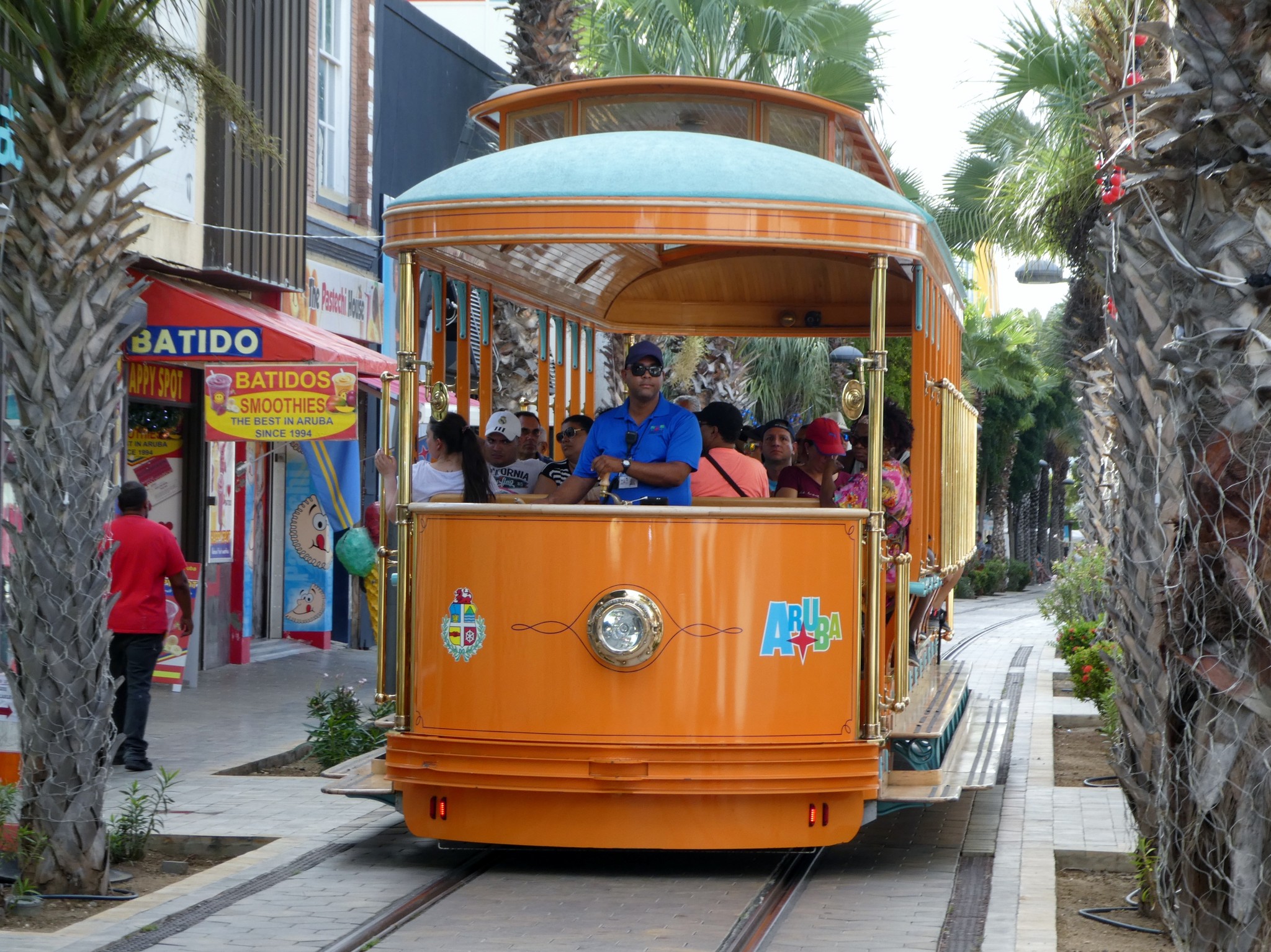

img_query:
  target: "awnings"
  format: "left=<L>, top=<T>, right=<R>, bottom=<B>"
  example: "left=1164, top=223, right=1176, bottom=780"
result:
left=120, top=265, right=402, bottom=378
left=358, top=376, right=480, bottom=427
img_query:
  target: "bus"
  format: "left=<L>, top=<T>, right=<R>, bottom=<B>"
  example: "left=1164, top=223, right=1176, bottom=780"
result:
left=379, top=75, right=976, bottom=872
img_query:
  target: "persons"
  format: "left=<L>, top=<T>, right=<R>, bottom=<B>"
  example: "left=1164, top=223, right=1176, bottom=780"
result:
left=1035, top=547, right=1051, bottom=585
left=964, top=531, right=987, bottom=570
left=374, top=412, right=499, bottom=524
left=476, top=396, right=951, bottom=669
left=529, top=340, right=703, bottom=507
left=1063, top=541, right=1090, bottom=574
left=95, top=481, right=195, bottom=771
left=985, top=535, right=993, bottom=544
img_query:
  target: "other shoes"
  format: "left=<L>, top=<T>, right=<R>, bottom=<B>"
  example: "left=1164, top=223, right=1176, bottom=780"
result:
left=920, top=609, right=952, bottom=640
left=98, top=755, right=126, bottom=765
left=125, top=758, right=153, bottom=770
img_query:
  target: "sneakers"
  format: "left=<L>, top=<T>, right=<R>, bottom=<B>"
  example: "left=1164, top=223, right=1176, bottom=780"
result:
left=908, top=637, right=920, bottom=668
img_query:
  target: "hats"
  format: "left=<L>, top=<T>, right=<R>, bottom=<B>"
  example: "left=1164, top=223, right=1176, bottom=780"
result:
left=821, top=412, right=851, bottom=432
left=760, top=418, right=795, bottom=442
left=623, top=340, right=664, bottom=369
left=692, top=401, right=744, bottom=431
left=805, top=417, right=847, bottom=456
left=857, top=414, right=869, bottom=425
left=745, top=424, right=765, bottom=441
left=484, top=410, right=522, bottom=441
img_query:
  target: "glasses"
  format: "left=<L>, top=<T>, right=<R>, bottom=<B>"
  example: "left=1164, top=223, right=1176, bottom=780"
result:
left=849, top=434, right=868, bottom=448
left=628, top=364, right=663, bottom=377
left=556, top=428, right=586, bottom=444
left=840, top=432, right=850, bottom=441
left=742, top=442, right=760, bottom=451
left=519, top=427, right=541, bottom=437
left=698, top=420, right=708, bottom=427
